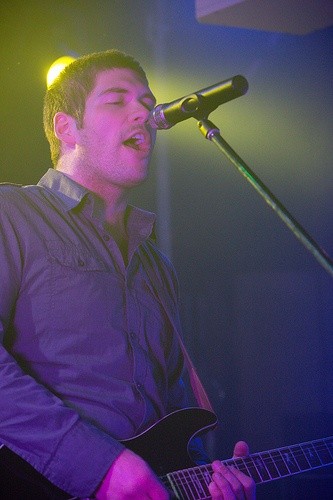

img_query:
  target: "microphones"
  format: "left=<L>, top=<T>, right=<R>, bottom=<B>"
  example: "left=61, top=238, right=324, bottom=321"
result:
left=145, top=74, right=249, bottom=132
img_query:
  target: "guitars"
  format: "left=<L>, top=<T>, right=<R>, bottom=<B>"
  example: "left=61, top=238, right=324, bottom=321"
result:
left=0, top=406, right=333, bottom=500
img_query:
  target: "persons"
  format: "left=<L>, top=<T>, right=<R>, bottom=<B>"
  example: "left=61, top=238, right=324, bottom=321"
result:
left=0, top=49, right=255, bottom=500
